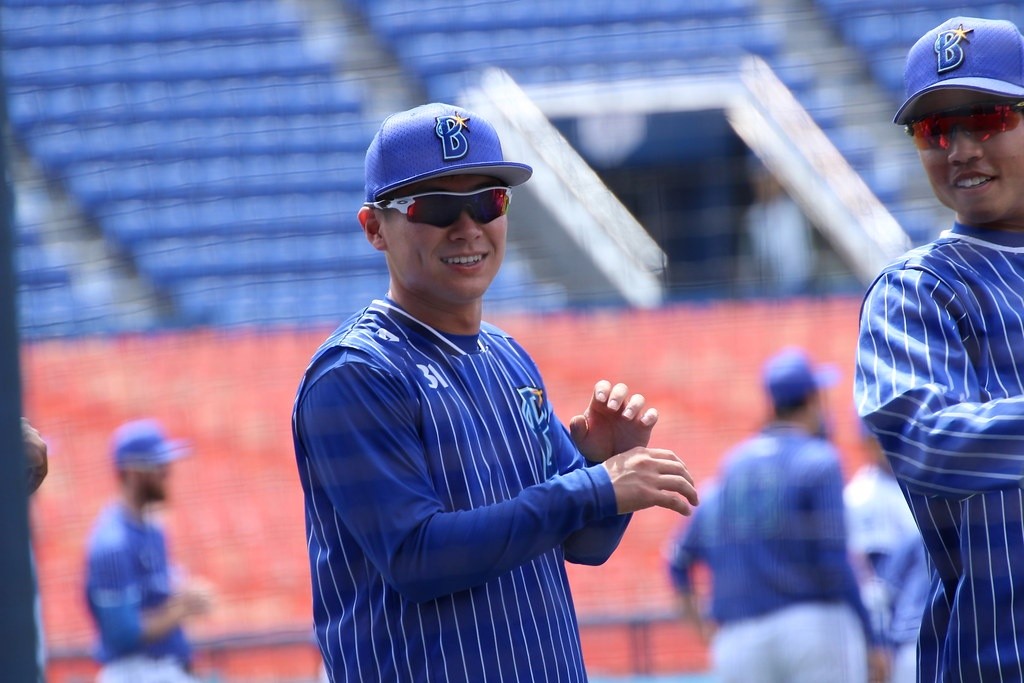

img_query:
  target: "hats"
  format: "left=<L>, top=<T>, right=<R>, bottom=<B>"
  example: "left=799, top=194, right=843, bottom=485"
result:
left=893, top=17, right=1024, bottom=125
left=110, top=422, right=185, bottom=468
left=364, top=102, right=533, bottom=203
left=766, top=353, right=839, bottom=400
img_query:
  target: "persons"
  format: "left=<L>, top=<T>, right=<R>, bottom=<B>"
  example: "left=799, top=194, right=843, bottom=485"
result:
left=291, top=102, right=700, bottom=683
left=853, top=15, right=1024, bottom=683
left=665, top=347, right=930, bottom=683
left=21, top=415, right=49, bottom=494
left=83, top=421, right=218, bottom=683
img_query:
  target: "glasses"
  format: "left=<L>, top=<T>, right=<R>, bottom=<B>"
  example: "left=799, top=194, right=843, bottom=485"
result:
left=907, top=99, right=1024, bottom=150
left=364, top=187, right=512, bottom=228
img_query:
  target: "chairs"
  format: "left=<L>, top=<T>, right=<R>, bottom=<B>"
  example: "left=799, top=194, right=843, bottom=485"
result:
left=0, top=0, right=1024, bottom=339
